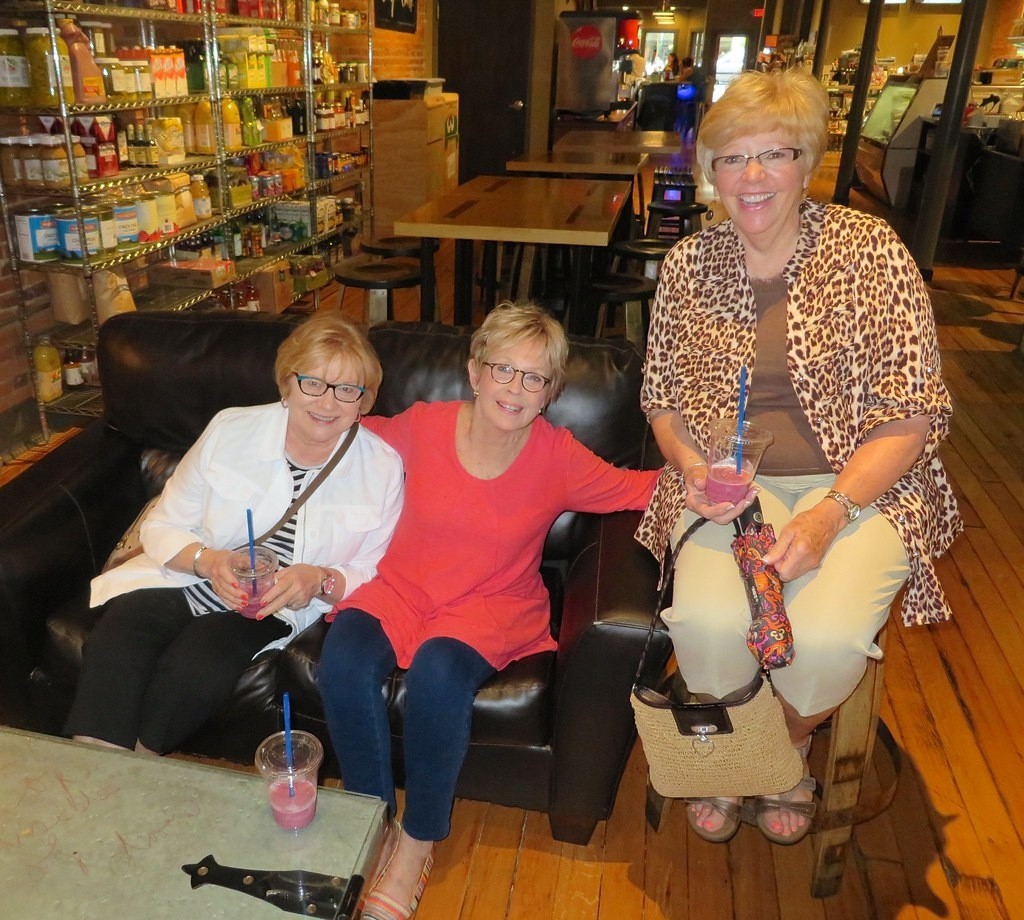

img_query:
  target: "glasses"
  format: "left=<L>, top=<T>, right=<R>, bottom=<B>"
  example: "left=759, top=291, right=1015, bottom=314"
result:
left=293, top=371, right=366, bottom=404
left=710, top=148, right=805, bottom=173
left=483, top=361, right=551, bottom=394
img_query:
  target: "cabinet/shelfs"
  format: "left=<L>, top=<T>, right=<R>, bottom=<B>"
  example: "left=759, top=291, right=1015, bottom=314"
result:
left=0, top=0, right=375, bottom=444
left=824, top=49, right=883, bottom=154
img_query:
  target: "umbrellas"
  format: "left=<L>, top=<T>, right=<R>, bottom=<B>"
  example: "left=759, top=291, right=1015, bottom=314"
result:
left=733, top=495, right=796, bottom=672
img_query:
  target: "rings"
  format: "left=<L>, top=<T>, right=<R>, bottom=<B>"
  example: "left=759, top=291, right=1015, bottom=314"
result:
left=286, top=603, right=292, bottom=608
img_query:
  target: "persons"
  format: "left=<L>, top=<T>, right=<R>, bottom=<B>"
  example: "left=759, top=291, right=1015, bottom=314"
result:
left=641, top=68, right=950, bottom=844
left=62, top=313, right=405, bottom=768
left=319, top=302, right=664, bottom=920
left=680, top=58, right=702, bottom=85
left=664, top=54, right=680, bottom=80
left=646, top=49, right=664, bottom=75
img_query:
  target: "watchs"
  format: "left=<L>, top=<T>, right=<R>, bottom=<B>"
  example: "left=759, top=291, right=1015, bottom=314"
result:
left=313, top=566, right=334, bottom=599
left=824, top=491, right=861, bottom=525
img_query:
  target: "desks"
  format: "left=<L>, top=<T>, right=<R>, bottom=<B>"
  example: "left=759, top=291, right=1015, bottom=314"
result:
left=0, top=727, right=389, bottom=920
left=394, top=130, right=682, bottom=339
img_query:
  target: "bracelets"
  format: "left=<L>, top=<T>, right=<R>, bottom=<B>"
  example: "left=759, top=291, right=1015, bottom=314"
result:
left=681, top=462, right=708, bottom=490
left=193, top=546, right=208, bottom=579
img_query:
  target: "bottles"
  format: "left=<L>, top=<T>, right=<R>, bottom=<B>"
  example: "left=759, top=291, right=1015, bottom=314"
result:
left=0, top=0, right=372, bottom=264
left=189, top=225, right=362, bottom=314
left=34, top=336, right=100, bottom=403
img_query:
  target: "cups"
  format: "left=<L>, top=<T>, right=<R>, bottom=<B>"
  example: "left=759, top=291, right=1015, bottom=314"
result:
left=229, top=546, right=280, bottom=620
left=256, top=730, right=324, bottom=830
left=705, top=417, right=773, bottom=504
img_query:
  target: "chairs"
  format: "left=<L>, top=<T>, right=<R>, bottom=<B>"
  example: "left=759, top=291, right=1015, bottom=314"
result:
left=908, top=115, right=1024, bottom=270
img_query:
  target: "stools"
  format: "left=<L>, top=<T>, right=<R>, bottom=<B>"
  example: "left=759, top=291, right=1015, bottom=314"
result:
left=652, top=176, right=697, bottom=200
left=615, top=237, right=677, bottom=276
left=646, top=200, right=708, bottom=239
left=654, top=165, right=694, bottom=178
left=561, top=272, right=658, bottom=352
left=645, top=624, right=901, bottom=898
left=330, top=231, right=442, bottom=327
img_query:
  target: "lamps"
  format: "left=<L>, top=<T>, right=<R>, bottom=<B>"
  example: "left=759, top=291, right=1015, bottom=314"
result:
left=652, top=0, right=675, bottom=24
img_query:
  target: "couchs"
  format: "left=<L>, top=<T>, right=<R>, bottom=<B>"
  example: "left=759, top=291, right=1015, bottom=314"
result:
left=0, top=312, right=675, bottom=846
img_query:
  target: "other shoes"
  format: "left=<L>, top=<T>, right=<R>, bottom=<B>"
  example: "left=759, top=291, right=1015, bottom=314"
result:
left=360, top=820, right=432, bottom=920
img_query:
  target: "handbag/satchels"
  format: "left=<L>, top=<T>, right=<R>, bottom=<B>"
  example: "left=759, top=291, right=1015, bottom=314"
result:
left=677, top=83, right=696, bottom=100
left=627, top=667, right=803, bottom=797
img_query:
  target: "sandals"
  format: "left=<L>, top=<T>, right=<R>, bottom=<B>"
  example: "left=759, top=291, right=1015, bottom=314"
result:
left=685, top=732, right=817, bottom=846
